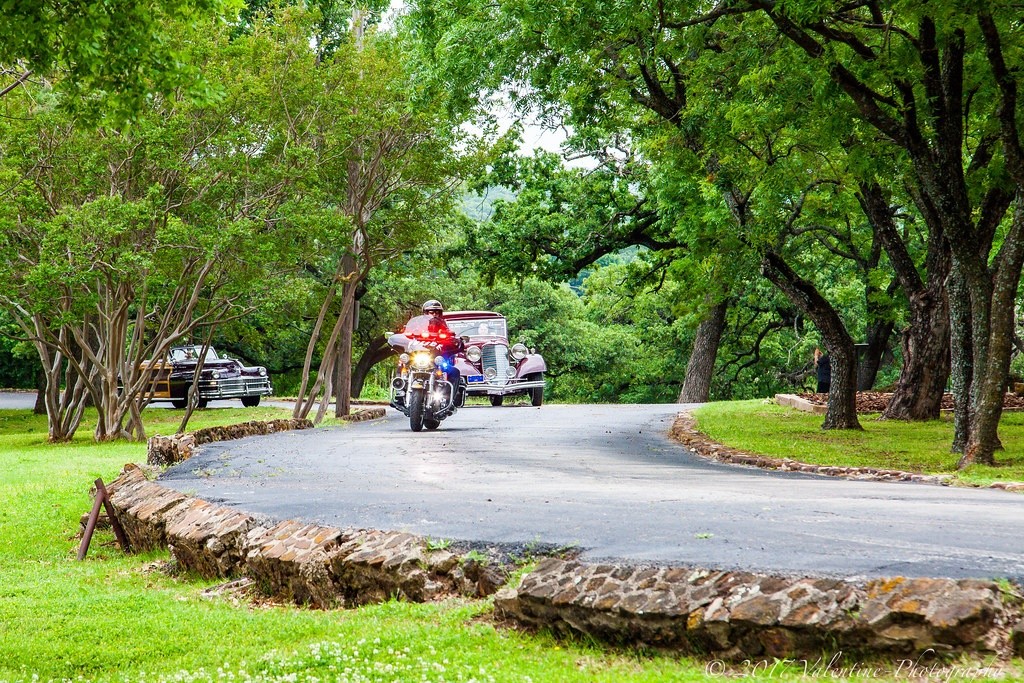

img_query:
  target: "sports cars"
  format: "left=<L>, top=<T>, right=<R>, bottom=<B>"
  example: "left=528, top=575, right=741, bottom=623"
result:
left=117, top=346, right=273, bottom=408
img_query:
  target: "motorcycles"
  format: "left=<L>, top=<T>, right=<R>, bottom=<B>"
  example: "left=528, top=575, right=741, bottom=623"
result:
left=384, top=299, right=470, bottom=431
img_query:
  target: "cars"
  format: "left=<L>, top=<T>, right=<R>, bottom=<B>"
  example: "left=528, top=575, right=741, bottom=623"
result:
left=443, top=311, right=547, bottom=408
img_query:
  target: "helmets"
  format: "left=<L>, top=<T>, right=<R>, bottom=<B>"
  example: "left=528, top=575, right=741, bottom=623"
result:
left=423, top=300, right=443, bottom=318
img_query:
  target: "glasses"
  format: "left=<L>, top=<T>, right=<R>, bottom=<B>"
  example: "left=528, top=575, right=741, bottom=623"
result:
left=429, top=310, right=439, bottom=314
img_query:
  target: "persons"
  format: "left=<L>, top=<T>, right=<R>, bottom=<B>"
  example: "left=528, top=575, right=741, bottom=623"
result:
left=405, top=299, right=464, bottom=410
left=478, top=323, right=488, bottom=335
